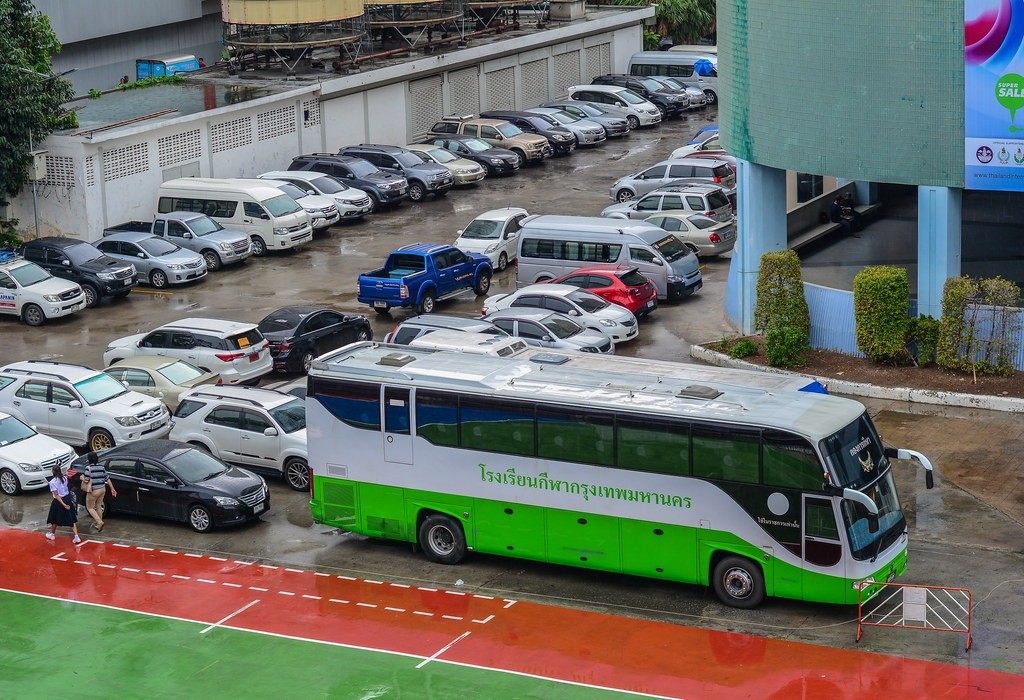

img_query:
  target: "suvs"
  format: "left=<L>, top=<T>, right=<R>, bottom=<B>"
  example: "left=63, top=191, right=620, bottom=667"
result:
left=0, top=360, right=175, bottom=452
left=385, top=313, right=510, bottom=350
left=103, top=318, right=274, bottom=386
left=168, top=382, right=312, bottom=491
left=0, top=144, right=452, bottom=326
left=601, top=126, right=738, bottom=224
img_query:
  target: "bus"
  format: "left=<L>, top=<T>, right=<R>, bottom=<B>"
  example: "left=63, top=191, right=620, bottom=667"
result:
left=306, top=329, right=934, bottom=609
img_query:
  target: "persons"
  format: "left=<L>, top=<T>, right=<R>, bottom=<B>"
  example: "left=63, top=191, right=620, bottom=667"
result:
left=198, top=58, right=206, bottom=68
left=45, top=465, right=81, bottom=543
left=830, top=191, right=863, bottom=238
left=80, top=452, right=117, bottom=532
left=119, top=75, right=128, bottom=85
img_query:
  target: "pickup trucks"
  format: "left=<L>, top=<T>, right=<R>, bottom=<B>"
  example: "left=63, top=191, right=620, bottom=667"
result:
left=357, top=242, right=493, bottom=315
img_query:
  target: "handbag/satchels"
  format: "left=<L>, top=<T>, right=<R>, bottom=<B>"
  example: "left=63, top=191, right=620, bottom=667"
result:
left=81, top=465, right=93, bottom=493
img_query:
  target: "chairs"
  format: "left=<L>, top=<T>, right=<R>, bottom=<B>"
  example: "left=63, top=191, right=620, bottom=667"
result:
left=359, top=411, right=769, bottom=483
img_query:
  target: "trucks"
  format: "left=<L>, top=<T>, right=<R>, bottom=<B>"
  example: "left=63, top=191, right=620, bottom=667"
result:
left=136, top=53, right=200, bottom=81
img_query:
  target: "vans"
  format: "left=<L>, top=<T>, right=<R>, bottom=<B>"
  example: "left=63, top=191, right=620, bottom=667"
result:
left=515, top=215, right=703, bottom=304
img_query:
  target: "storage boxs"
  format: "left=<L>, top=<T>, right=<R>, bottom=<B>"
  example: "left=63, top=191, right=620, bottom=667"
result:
left=389, top=269, right=415, bottom=279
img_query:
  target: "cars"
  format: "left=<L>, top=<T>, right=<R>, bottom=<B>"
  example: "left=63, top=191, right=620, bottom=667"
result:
left=406, top=46, right=718, bottom=186
left=0, top=411, right=80, bottom=496
left=453, top=208, right=529, bottom=270
left=642, top=212, right=737, bottom=260
left=475, top=307, right=616, bottom=356
left=255, top=306, right=372, bottom=376
left=482, top=283, right=639, bottom=344
left=100, top=357, right=221, bottom=417
left=536, top=265, right=658, bottom=318
left=66, top=438, right=270, bottom=533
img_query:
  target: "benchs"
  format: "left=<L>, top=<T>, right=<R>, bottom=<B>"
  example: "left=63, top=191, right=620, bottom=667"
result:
left=789, top=201, right=883, bottom=250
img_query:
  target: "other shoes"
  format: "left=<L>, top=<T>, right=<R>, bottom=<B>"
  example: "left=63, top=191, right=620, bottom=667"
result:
left=95, top=521, right=104, bottom=532
left=72, top=537, right=81, bottom=544
left=45, top=532, right=55, bottom=540
